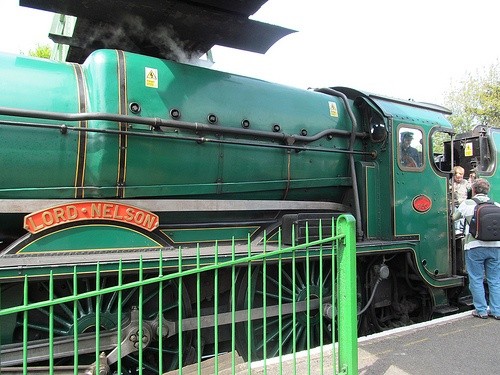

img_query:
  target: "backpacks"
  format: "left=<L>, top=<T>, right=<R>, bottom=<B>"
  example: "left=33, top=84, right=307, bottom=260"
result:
left=470, top=198, right=500, bottom=241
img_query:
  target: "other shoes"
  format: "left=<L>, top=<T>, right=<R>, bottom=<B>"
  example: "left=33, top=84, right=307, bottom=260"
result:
left=471, top=310, right=488, bottom=319
left=455, top=232, right=465, bottom=238
left=488, top=312, right=500, bottom=320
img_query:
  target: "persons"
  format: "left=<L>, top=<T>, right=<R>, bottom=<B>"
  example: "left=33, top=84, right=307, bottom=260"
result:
left=452, top=178, right=500, bottom=320
left=398, top=131, right=419, bottom=167
left=449, top=166, right=474, bottom=235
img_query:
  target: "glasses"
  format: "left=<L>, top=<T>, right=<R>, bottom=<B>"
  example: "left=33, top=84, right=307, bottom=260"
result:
left=470, top=164, right=477, bottom=167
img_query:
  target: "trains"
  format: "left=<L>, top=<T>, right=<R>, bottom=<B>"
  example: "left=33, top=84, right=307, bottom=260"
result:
left=0, top=49, right=500, bottom=375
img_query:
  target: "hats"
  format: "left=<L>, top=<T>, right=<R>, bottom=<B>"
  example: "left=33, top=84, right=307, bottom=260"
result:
left=469, top=158, right=479, bottom=163
left=400, top=131, right=413, bottom=139
